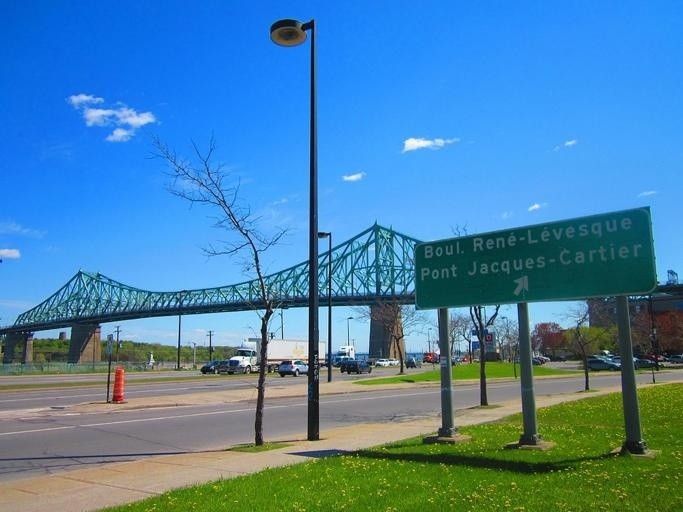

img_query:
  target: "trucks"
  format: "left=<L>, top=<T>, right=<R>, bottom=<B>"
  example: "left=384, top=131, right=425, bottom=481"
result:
left=226, top=338, right=326, bottom=374
left=335, top=346, right=354, bottom=363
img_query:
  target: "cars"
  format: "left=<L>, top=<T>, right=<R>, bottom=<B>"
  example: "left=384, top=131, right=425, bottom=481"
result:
left=201, top=360, right=228, bottom=374
left=341, top=360, right=372, bottom=374
left=559, top=355, right=567, bottom=362
left=404, top=356, right=422, bottom=368
left=452, top=356, right=467, bottom=366
left=375, top=358, right=399, bottom=367
left=532, top=356, right=550, bottom=364
left=586, top=350, right=683, bottom=371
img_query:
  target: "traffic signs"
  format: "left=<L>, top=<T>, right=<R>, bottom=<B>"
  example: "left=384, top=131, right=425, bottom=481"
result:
left=413, top=206, right=657, bottom=310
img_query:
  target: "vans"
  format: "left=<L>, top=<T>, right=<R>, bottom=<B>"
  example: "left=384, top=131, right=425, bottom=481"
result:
left=423, top=352, right=437, bottom=363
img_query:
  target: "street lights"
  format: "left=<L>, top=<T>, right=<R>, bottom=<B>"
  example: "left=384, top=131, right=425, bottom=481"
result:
left=270, top=17, right=331, bottom=441
left=426, top=328, right=433, bottom=352
left=177, top=290, right=188, bottom=370
left=502, top=316, right=510, bottom=336
left=347, top=316, right=354, bottom=345
